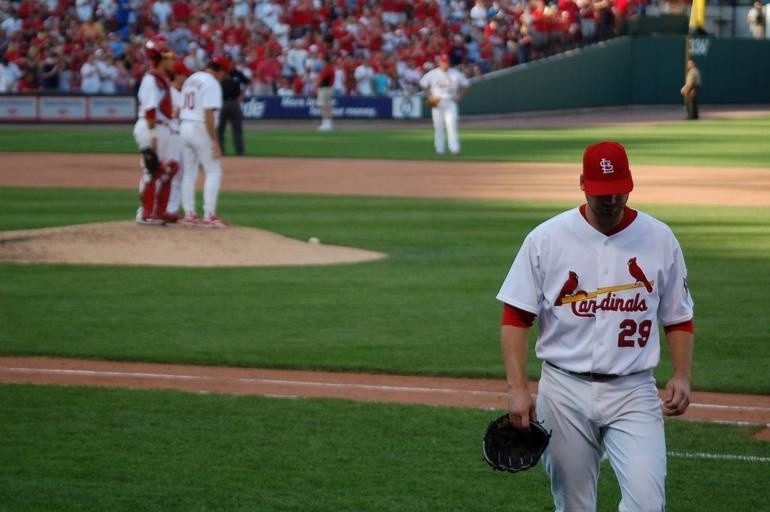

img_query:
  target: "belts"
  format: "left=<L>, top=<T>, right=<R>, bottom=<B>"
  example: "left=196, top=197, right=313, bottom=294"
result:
left=545, top=360, right=646, bottom=378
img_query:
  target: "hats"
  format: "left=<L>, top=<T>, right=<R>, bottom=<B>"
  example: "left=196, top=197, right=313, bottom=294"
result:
left=582, top=141, right=632, bottom=196
left=145, top=37, right=232, bottom=73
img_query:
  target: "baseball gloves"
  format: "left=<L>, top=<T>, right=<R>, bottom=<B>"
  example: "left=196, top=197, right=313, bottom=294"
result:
left=482, top=409, right=552, bottom=474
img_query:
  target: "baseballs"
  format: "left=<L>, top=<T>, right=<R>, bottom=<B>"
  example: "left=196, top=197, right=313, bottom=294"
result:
left=308, top=236, right=318, bottom=244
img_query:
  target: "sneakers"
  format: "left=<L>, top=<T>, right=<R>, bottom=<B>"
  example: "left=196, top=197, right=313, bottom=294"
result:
left=136, top=212, right=230, bottom=230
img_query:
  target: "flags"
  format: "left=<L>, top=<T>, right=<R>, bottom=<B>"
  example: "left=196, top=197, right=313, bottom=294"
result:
left=688, top=0, right=706, bottom=33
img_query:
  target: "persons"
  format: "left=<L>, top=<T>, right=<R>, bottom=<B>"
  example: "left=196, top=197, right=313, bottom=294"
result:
left=132, top=34, right=253, bottom=230
left=312, top=51, right=335, bottom=131
left=680, top=57, right=703, bottom=120
left=747, top=0, right=767, bottom=40
left=417, top=55, right=470, bottom=156
left=494, top=137, right=699, bottom=512
